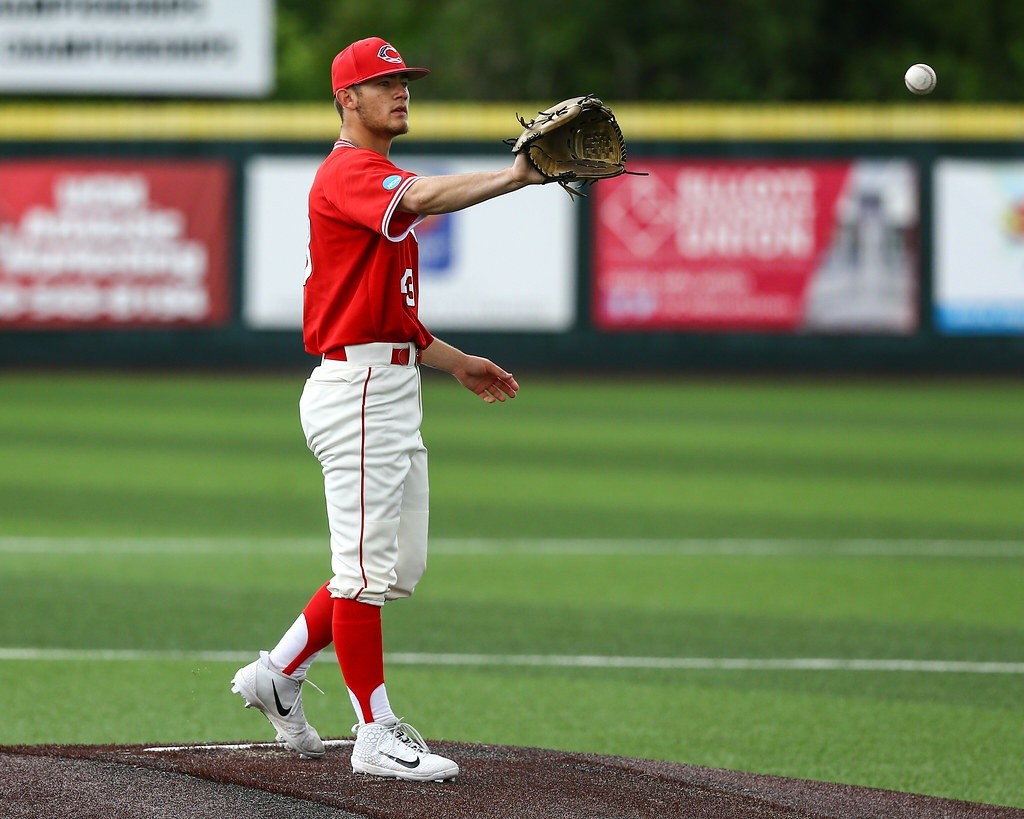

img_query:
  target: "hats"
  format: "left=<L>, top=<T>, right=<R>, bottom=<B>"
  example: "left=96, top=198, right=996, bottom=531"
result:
left=332, top=37, right=431, bottom=98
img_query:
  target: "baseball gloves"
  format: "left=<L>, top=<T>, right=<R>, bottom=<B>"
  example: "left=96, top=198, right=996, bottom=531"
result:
left=502, top=91, right=651, bottom=204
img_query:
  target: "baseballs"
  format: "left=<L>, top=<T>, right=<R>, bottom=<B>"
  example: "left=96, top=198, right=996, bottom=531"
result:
left=903, top=62, right=937, bottom=96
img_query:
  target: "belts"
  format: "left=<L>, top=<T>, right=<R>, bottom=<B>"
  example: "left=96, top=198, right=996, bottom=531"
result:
left=324, top=345, right=423, bottom=366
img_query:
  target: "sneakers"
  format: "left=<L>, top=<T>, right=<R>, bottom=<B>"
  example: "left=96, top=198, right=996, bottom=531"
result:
left=351, top=717, right=459, bottom=783
left=230, top=651, right=325, bottom=758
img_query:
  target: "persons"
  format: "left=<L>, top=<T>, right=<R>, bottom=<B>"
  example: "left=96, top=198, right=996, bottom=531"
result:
left=230, top=38, right=557, bottom=783
